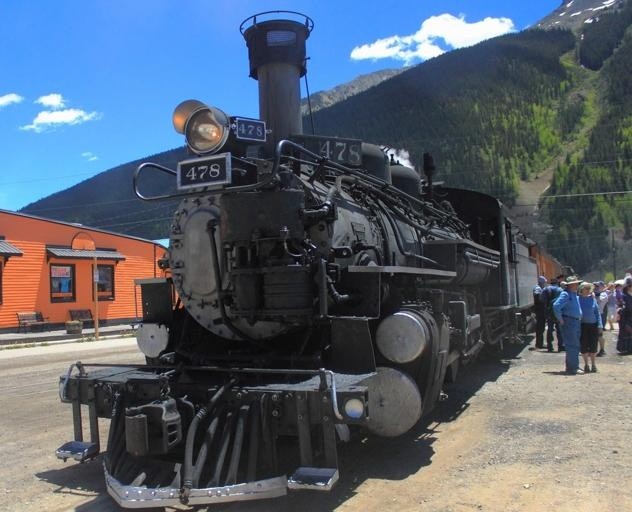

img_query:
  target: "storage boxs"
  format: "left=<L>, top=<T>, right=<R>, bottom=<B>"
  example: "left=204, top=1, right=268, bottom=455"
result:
left=69, top=308, right=101, bottom=329
left=16, top=311, right=52, bottom=333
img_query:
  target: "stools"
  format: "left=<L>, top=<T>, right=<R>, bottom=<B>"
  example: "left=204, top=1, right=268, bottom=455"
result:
left=561, top=313, right=583, bottom=321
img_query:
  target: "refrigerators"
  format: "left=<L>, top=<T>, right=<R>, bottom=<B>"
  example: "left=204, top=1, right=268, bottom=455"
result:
left=596, top=349, right=606, bottom=357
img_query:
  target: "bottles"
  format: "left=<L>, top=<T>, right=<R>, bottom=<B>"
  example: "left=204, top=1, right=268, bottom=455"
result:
left=550, top=279, right=560, bottom=284
left=579, top=281, right=592, bottom=291
left=563, top=276, right=583, bottom=287
left=622, top=277, right=632, bottom=291
left=593, top=281, right=604, bottom=288
left=539, top=276, right=547, bottom=284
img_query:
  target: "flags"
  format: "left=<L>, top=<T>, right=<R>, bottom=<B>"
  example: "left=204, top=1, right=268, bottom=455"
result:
left=65, top=320, right=84, bottom=333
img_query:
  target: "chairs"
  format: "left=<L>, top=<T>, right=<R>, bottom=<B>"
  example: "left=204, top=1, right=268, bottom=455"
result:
left=591, top=365, right=597, bottom=372
left=584, top=365, right=590, bottom=373
left=569, top=368, right=584, bottom=375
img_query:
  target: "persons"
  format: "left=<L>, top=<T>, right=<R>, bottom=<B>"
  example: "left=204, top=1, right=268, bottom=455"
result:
left=530, top=271, right=632, bottom=377
